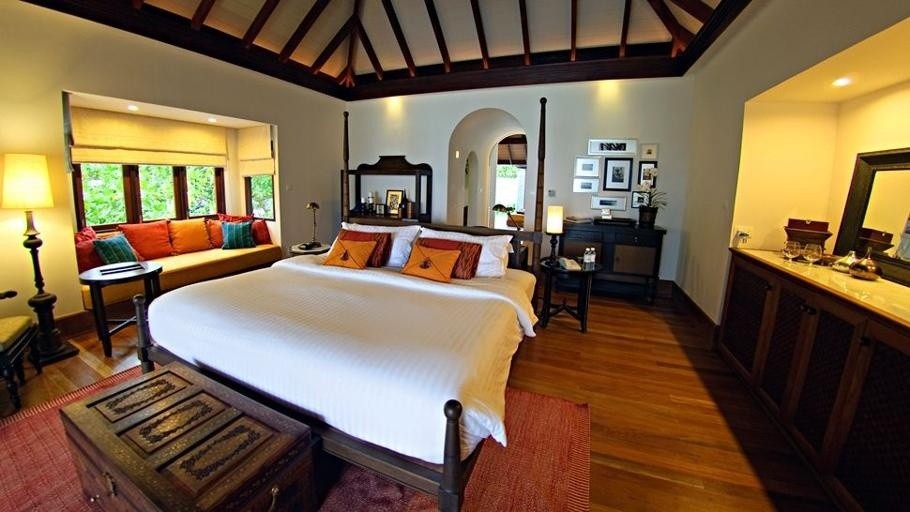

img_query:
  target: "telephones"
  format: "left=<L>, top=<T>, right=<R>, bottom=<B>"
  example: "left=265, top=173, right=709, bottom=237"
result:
left=558, top=257, right=582, bottom=271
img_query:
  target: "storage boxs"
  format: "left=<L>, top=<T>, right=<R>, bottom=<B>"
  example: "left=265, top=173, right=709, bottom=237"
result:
left=54, top=355, right=321, bottom=512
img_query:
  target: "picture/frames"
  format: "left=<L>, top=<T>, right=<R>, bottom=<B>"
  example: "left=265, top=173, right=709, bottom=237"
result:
left=573, top=178, right=599, bottom=193
left=588, top=138, right=637, bottom=156
left=603, top=157, right=634, bottom=192
left=385, top=190, right=403, bottom=216
left=589, top=195, right=627, bottom=212
left=573, top=155, right=598, bottom=176
left=637, top=161, right=658, bottom=189
left=639, top=142, right=659, bottom=161
left=631, top=191, right=652, bottom=209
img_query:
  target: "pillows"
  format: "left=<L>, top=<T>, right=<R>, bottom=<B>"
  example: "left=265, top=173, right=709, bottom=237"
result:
left=319, top=220, right=516, bottom=285
left=72, top=226, right=140, bottom=278
left=160, top=216, right=217, bottom=256
left=203, top=212, right=274, bottom=252
left=115, top=220, right=177, bottom=262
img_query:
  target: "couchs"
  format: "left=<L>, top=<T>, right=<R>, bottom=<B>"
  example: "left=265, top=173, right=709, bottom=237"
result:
left=71, top=215, right=284, bottom=314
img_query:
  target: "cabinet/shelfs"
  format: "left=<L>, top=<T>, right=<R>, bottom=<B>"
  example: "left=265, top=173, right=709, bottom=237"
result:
left=550, top=216, right=668, bottom=311
left=820, top=282, right=910, bottom=511
left=754, top=256, right=893, bottom=473
left=719, top=246, right=838, bottom=400
left=337, top=153, right=432, bottom=229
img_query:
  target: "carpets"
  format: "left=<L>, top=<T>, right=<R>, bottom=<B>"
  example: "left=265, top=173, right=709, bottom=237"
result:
left=1, top=348, right=594, bottom=511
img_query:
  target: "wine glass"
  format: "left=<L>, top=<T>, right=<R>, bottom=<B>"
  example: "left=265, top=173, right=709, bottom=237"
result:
left=804, top=243, right=823, bottom=268
left=781, top=240, right=800, bottom=264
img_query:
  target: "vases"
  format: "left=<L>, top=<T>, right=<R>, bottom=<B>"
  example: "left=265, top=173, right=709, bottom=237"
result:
left=638, top=203, right=659, bottom=231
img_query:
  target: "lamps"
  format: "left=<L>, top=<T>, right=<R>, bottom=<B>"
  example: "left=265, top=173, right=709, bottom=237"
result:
left=491, top=201, right=525, bottom=256
left=1, top=151, right=78, bottom=368
left=541, top=200, right=564, bottom=269
left=304, top=201, right=320, bottom=242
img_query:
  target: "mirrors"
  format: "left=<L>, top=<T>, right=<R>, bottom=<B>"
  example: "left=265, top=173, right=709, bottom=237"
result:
left=831, top=145, right=909, bottom=289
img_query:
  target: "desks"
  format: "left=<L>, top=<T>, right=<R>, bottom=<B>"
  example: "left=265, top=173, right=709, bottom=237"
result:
left=534, top=252, right=604, bottom=336
left=506, top=211, right=524, bottom=227
left=76, top=260, right=166, bottom=360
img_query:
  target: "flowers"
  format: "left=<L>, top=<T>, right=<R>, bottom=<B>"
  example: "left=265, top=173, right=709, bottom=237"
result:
left=631, top=166, right=670, bottom=213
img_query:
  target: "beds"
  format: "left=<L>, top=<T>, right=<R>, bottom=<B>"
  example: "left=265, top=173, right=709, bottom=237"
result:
left=121, top=88, right=561, bottom=512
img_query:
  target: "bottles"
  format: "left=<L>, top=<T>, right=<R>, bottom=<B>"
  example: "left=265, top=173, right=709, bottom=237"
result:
left=832, top=247, right=882, bottom=280
left=406, top=202, right=416, bottom=219
left=582, top=248, right=596, bottom=266
left=399, top=188, right=406, bottom=220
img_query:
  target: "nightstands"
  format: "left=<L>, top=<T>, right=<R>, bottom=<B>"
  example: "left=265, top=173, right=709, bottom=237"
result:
left=287, top=241, right=332, bottom=259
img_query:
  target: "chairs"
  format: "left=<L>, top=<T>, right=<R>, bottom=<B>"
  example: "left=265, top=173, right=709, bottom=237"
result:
left=0, top=290, right=43, bottom=414
